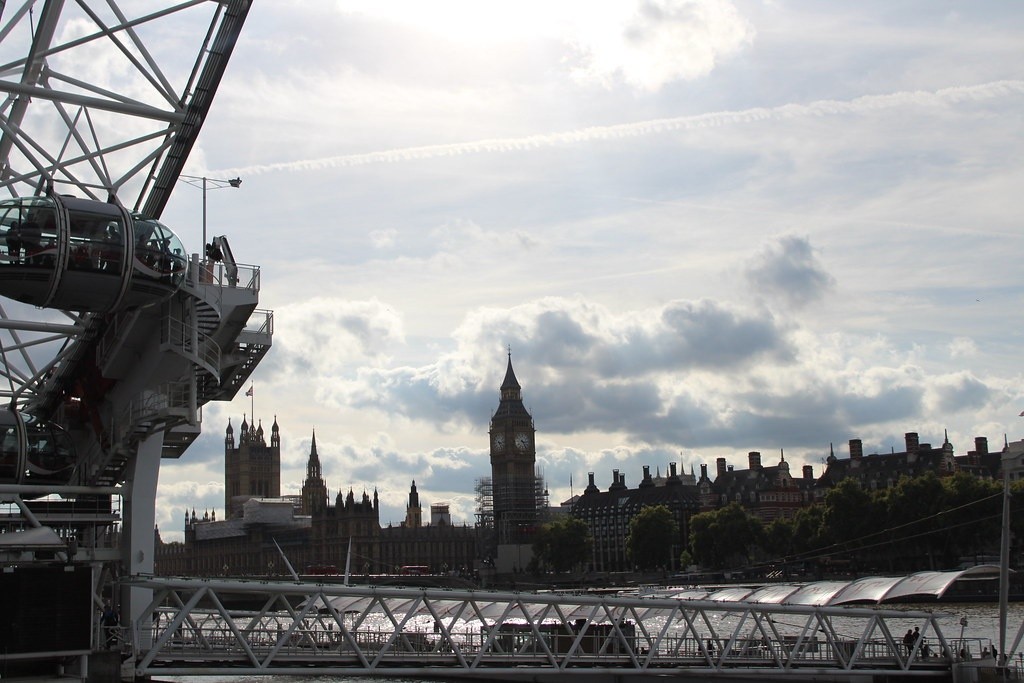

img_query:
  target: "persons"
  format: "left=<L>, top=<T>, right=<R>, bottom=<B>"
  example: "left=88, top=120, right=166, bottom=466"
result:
left=100, top=226, right=182, bottom=283
left=982, top=645, right=998, bottom=661
left=903, top=627, right=933, bottom=657
left=5, top=214, right=56, bottom=265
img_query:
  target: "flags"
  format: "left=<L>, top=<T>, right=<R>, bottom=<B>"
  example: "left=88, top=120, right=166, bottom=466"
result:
left=246, top=386, right=253, bottom=396
